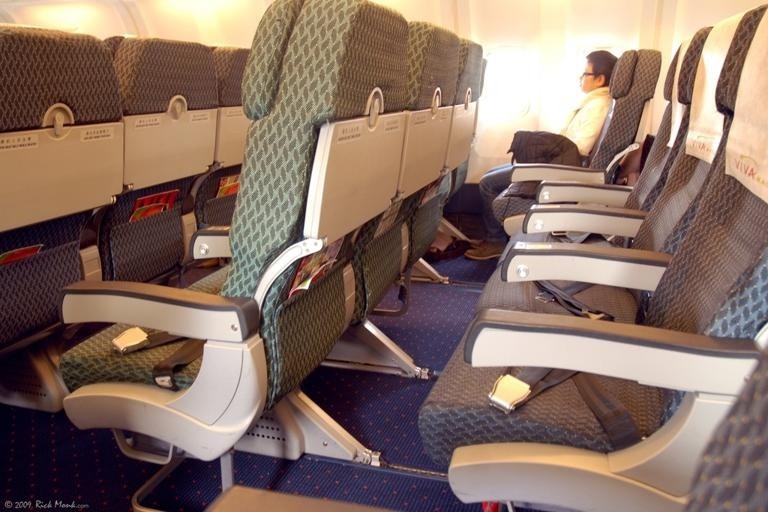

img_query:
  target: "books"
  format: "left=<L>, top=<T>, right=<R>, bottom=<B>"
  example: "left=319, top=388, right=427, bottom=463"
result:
left=420, top=171, right=452, bottom=209
left=372, top=200, right=400, bottom=239
left=289, top=238, right=344, bottom=290
left=216, top=181, right=242, bottom=198
left=128, top=203, right=165, bottom=224
left=0, top=243, right=45, bottom=265
left=288, top=258, right=337, bottom=299
left=219, top=173, right=241, bottom=186
left=133, top=189, right=179, bottom=211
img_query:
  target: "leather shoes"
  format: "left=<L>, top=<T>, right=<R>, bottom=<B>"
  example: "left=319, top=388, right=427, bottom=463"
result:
left=465, top=241, right=503, bottom=261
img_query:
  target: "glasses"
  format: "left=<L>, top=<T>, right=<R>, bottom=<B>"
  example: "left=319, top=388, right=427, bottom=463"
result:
left=582, top=72, right=594, bottom=77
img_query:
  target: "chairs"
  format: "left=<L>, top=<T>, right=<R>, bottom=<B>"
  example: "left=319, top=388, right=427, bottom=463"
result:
left=0, top=0, right=768, bottom=512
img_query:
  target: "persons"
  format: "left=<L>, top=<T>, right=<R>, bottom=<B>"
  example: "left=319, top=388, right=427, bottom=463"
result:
left=462, top=49, right=619, bottom=263
